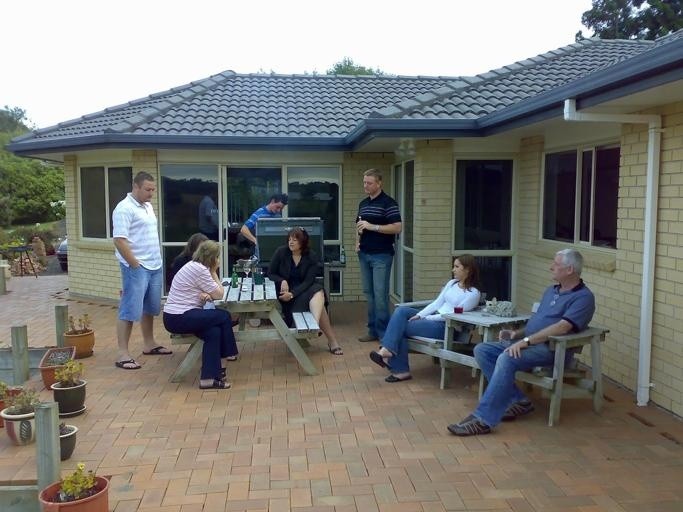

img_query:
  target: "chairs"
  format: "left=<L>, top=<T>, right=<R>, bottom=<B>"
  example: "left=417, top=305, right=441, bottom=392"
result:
left=394, top=295, right=610, bottom=427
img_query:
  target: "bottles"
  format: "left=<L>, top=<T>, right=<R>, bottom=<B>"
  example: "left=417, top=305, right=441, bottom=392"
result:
left=340, top=245, right=346, bottom=264
left=231, top=264, right=238, bottom=288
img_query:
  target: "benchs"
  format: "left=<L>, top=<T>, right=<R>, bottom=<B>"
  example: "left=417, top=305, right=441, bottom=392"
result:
left=172, top=311, right=321, bottom=342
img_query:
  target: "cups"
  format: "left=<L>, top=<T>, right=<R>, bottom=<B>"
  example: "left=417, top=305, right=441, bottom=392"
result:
left=453, top=307, right=462, bottom=313
left=255, top=268, right=263, bottom=285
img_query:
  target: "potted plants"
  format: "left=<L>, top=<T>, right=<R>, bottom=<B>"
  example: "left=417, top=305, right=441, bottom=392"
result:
left=0, top=313, right=110, bottom=512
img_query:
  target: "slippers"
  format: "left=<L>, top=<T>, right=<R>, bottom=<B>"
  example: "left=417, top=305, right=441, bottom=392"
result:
left=115, top=359, right=141, bottom=369
left=143, top=346, right=173, bottom=355
left=199, top=380, right=231, bottom=389
left=370, top=351, right=386, bottom=368
left=385, top=374, right=412, bottom=382
left=328, top=343, right=343, bottom=355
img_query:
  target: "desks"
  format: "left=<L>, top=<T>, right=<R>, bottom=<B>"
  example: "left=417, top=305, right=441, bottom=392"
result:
left=171, top=277, right=317, bottom=383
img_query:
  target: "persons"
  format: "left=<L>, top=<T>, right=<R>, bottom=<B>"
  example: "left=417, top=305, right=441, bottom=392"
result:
left=350, top=167, right=401, bottom=343
left=161, top=239, right=238, bottom=391
left=368, top=252, right=483, bottom=383
left=170, top=232, right=237, bottom=363
left=238, top=191, right=288, bottom=267
left=322, top=182, right=339, bottom=240
left=112, top=170, right=172, bottom=370
left=446, top=247, right=597, bottom=436
left=195, top=184, right=220, bottom=238
left=267, top=227, right=344, bottom=358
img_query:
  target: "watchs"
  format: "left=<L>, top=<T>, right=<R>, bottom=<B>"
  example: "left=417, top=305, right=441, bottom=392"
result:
left=288, top=293, right=293, bottom=299
left=520, top=336, right=533, bottom=347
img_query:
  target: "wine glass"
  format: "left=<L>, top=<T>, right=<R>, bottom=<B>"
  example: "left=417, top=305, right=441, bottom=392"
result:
left=242, top=260, right=252, bottom=290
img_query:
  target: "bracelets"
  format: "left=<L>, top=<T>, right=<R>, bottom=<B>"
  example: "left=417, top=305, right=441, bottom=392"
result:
left=372, top=223, right=381, bottom=233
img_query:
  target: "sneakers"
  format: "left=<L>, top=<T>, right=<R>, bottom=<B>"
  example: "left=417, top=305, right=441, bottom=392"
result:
left=358, top=334, right=374, bottom=342
left=502, top=400, right=535, bottom=420
left=447, top=414, right=491, bottom=435
